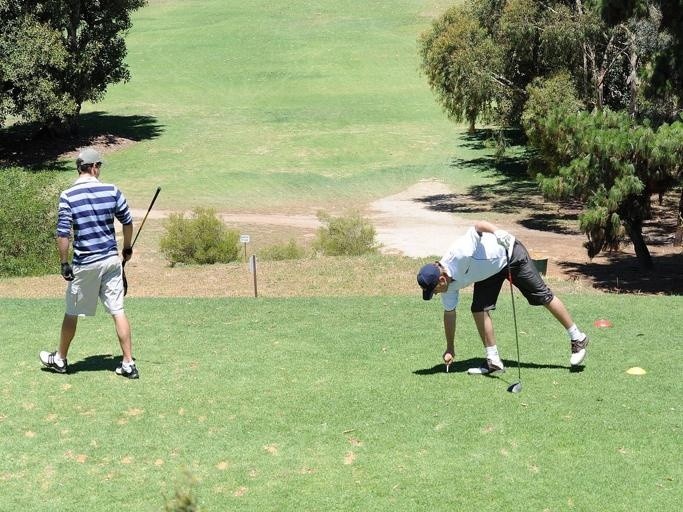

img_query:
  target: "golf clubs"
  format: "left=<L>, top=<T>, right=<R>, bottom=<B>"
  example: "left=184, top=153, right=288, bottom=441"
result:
left=122, top=187, right=162, bottom=297
left=506, top=247, right=522, bottom=394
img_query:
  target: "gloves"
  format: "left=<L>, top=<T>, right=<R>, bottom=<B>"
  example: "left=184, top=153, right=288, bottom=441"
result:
left=496, top=230, right=513, bottom=249
left=61, top=262, right=75, bottom=281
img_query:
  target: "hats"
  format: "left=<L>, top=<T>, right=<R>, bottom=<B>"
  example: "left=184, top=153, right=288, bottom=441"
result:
left=78, top=148, right=107, bottom=165
left=418, top=264, right=442, bottom=300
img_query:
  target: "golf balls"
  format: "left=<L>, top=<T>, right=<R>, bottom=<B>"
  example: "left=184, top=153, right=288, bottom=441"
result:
left=443, top=353, right=453, bottom=362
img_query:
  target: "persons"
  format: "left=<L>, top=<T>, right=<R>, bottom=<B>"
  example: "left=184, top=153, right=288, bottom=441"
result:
left=37, top=148, right=140, bottom=380
left=417, top=220, right=589, bottom=375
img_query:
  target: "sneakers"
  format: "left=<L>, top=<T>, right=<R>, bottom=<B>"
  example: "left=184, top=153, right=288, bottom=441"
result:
left=116, top=360, right=139, bottom=378
left=40, top=351, right=68, bottom=373
left=568, top=332, right=589, bottom=366
left=467, top=359, right=503, bottom=374
